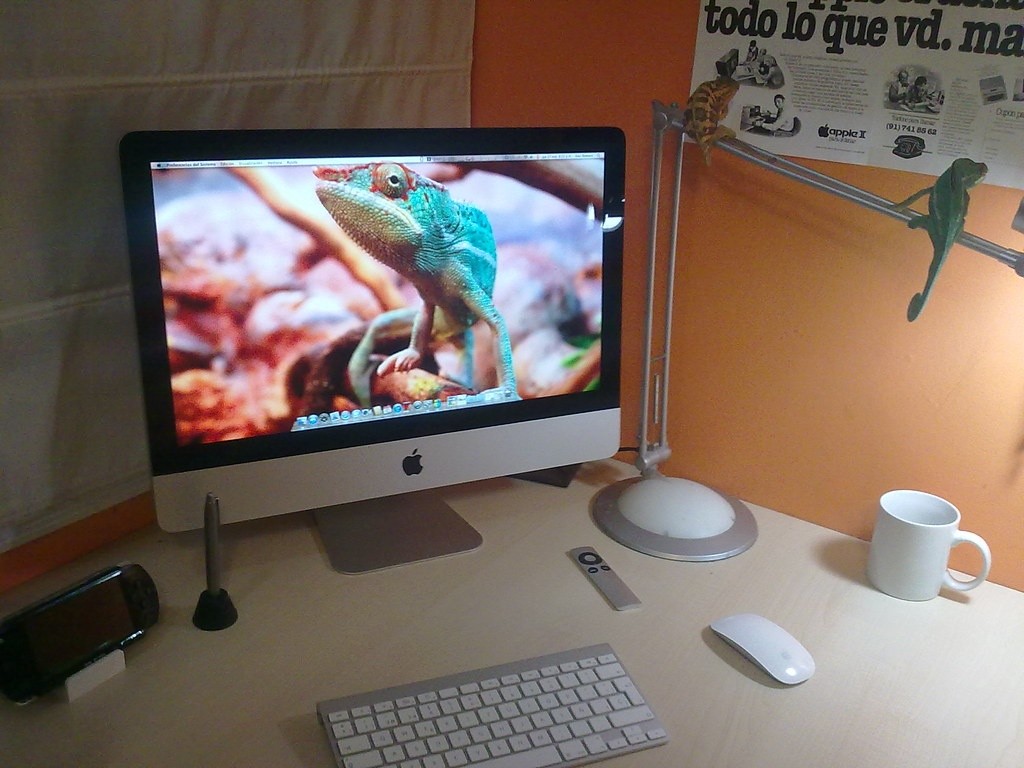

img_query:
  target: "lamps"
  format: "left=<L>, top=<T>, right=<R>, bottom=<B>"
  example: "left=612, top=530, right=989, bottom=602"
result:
left=585, top=97, right=1024, bottom=566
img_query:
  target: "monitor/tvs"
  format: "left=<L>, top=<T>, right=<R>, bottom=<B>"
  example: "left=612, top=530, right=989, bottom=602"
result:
left=121, top=126, right=626, bottom=576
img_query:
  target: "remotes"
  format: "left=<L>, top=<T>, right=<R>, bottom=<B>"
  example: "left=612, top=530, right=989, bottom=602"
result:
left=569, top=546, right=642, bottom=612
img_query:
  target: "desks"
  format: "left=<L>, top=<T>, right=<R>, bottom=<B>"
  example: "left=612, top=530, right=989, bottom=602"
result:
left=0, top=457, right=1024, bottom=768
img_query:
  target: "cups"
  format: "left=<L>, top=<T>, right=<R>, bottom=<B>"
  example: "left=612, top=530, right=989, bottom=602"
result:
left=865, top=489, right=992, bottom=601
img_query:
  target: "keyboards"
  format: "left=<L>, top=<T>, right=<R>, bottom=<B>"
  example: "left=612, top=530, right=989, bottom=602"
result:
left=316, top=645, right=670, bottom=768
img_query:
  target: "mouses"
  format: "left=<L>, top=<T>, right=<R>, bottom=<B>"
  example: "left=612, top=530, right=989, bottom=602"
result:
left=709, top=613, right=815, bottom=686
